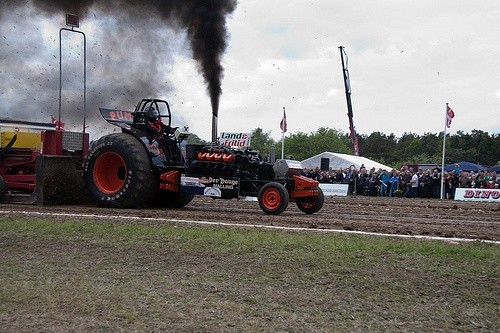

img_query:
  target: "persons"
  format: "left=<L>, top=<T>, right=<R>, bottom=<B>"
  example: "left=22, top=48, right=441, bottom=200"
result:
left=301, top=163, right=500, bottom=200
left=145, top=107, right=184, bottom=165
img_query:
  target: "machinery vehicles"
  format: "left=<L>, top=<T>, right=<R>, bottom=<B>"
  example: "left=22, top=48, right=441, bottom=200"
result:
left=83, top=98, right=325, bottom=215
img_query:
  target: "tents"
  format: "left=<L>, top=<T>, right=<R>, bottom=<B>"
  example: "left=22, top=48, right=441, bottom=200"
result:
left=444, top=161, right=488, bottom=172
left=488, top=165, right=500, bottom=174
left=300, top=152, right=399, bottom=173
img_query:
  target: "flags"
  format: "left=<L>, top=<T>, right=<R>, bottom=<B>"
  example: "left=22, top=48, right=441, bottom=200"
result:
left=280, top=110, right=287, bottom=133
left=446, top=106, right=454, bottom=128
